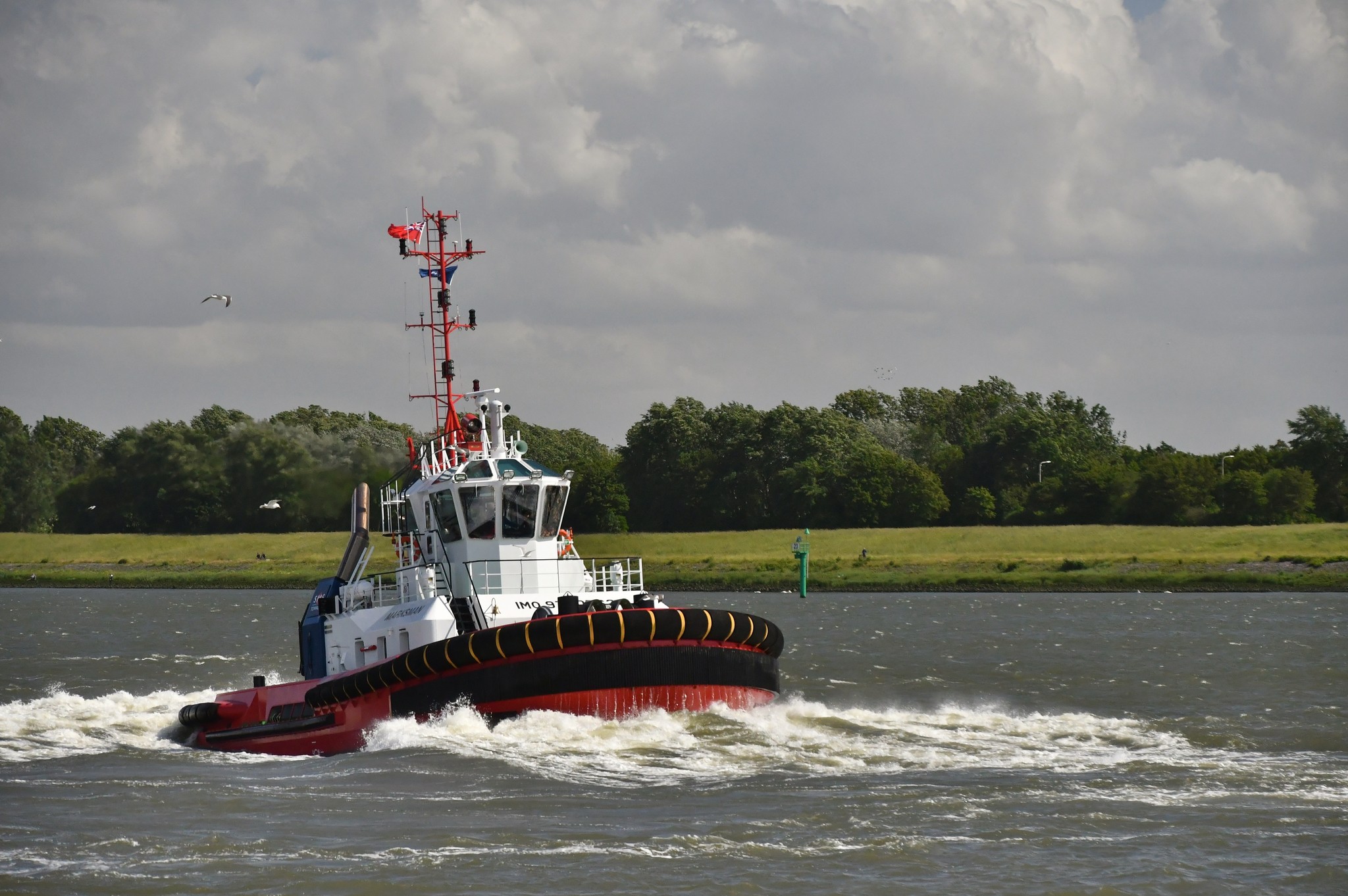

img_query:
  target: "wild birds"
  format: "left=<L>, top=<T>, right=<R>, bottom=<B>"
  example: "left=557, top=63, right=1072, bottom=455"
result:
left=87, top=505, right=96, bottom=510
left=1159, top=589, right=1173, bottom=594
left=873, top=366, right=897, bottom=381
left=512, top=544, right=537, bottom=558
left=259, top=499, right=282, bottom=509
left=199, top=293, right=232, bottom=309
left=1136, top=589, right=1141, bottom=594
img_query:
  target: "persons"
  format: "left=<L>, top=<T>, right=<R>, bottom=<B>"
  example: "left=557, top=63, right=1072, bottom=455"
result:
left=31, top=573, right=36, bottom=580
left=256, top=552, right=265, bottom=560
left=862, top=548, right=866, bottom=557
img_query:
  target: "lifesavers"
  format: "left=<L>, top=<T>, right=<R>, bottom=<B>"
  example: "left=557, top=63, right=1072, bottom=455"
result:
left=557, top=530, right=571, bottom=556
left=395, top=537, right=421, bottom=563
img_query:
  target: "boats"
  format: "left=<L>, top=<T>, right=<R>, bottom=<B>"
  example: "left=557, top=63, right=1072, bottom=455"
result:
left=176, top=194, right=784, bottom=759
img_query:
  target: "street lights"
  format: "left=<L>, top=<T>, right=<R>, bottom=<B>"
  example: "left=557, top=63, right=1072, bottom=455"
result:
left=1221, top=455, right=1235, bottom=478
left=1039, top=461, right=1051, bottom=483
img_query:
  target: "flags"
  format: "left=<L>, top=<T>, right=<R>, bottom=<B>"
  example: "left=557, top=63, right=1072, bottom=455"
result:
left=419, top=265, right=457, bottom=286
left=388, top=221, right=425, bottom=244
